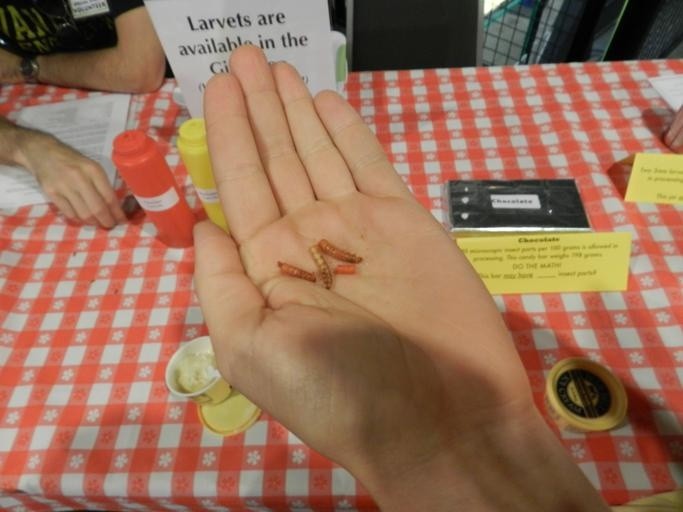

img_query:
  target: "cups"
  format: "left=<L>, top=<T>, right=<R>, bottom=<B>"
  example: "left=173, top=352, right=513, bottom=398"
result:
left=165, top=336, right=232, bottom=404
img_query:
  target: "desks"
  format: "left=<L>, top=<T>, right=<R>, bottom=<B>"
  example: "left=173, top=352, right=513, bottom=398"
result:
left=1, top=58, right=681, bottom=512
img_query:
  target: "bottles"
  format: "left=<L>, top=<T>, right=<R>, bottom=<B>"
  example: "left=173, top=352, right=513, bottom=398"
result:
left=177, top=118, right=232, bottom=235
left=112, top=130, right=198, bottom=247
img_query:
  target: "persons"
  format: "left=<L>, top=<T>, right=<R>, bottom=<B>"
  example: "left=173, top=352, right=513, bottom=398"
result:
left=0, top=0, right=167, bottom=229
left=190, top=44, right=615, bottom=510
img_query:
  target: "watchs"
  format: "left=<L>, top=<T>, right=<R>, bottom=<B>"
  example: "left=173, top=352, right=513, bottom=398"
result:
left=20, top=54, right=40, bottom=84
